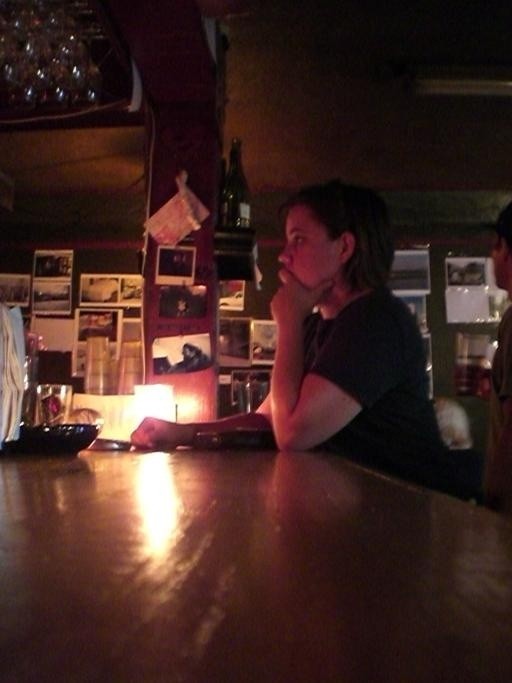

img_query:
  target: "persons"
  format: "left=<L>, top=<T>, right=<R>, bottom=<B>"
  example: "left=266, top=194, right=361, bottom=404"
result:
left=131, top=179, right=465, bottom=501
left=174, top=296, right=192, bottom=317
left=476, top=199, right=511, bottom=508
left=174, top=342, right=209, bottom=367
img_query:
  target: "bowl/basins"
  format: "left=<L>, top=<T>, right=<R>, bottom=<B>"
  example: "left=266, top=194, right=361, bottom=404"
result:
left=19, top=422, right=100, bottom=453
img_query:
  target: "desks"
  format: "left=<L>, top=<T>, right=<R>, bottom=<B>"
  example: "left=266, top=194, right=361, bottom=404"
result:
left=0, top=440, right=512, bottom=683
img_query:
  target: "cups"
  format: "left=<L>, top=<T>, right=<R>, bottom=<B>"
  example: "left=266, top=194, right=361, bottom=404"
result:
left=83, top=335, right=144, bottom=395
left=32, top=383, right=73, bottom=425
left=0, top=0, right=100, bottom=109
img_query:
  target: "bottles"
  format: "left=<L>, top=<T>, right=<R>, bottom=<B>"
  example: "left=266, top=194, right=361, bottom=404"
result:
left=221, top=137, right=252, bottom=228
